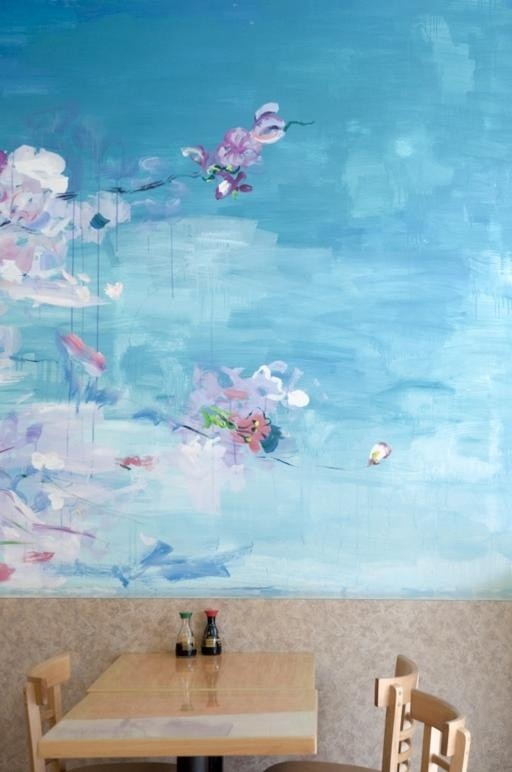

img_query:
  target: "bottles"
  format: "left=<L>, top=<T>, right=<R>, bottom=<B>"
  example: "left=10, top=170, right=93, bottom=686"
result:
left=177, top=609, right=221, bottom=659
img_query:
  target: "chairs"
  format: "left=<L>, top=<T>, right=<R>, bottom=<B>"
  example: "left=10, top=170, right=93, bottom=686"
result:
left=407, top=688, right=471, bottom=772
left=23, top=654, right=177, bottom=772
left=265, top=654, right=419, bottom=772
